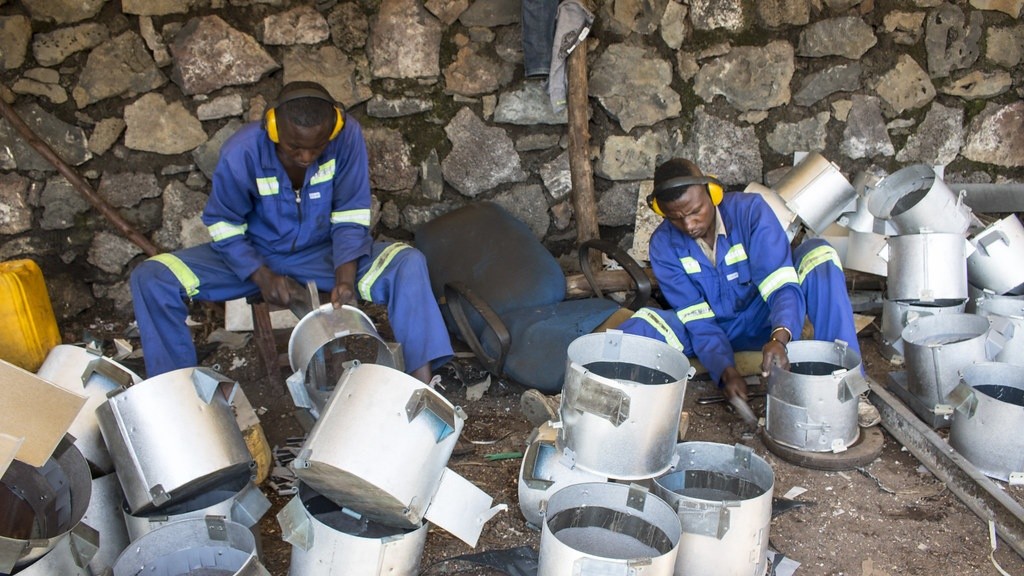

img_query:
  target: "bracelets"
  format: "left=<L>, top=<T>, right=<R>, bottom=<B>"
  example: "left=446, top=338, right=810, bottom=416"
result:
left=771, top=328, right=792, bottom=353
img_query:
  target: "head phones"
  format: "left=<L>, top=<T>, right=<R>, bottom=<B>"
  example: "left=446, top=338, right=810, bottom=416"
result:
left=646, top=174, right=724, bottom=218
left=264, top=88, right=347, bottom=143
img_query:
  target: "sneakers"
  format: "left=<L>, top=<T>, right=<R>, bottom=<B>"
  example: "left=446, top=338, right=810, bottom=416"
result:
left=521, top=388, right=561, bottom=427
left=425, top=375, right=476, bottom=453
left=857, top=393, right=882, bottom=428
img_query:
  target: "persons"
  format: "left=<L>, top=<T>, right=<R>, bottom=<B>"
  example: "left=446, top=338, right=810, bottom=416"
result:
left=128, top=82, right=475, bottom=454
left=617, top=159, right=881, bottom=427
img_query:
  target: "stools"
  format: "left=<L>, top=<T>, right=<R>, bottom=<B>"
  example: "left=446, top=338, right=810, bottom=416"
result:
left=245, top=286, right=346, bottom=398
